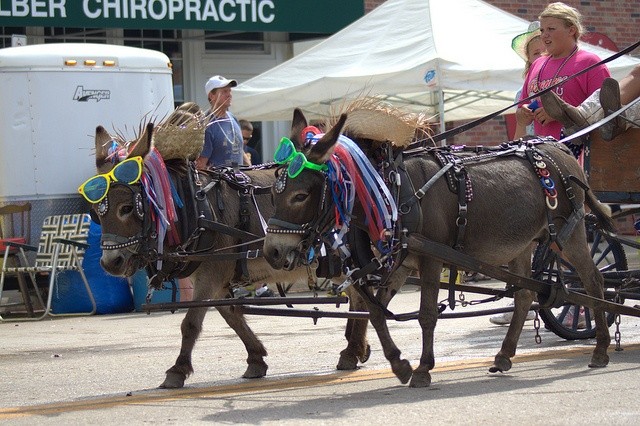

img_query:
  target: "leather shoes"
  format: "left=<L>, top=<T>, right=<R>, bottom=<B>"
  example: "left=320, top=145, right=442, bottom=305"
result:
left=255, top=289, right=279, bottom=297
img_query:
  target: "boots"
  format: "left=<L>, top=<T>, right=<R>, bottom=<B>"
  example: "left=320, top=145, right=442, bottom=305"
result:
left=540, top=88, right=604, bottom=146
left=600, top=78, right=640, bottom=141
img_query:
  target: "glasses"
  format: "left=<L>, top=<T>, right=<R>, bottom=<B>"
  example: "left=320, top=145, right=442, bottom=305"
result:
left=273, top=137, right=328, bottom=179
left=78, top=155, right=145, bottom=203
left=243, top=136, right=252, bottom=140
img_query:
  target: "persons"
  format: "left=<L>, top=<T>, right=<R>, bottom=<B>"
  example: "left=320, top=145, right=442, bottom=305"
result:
left=238, top=119, right=262, bottom=166
left=194, top=75, right=253, bottom=172
left=517, top=2, right=613, bottom=219
left=540, top=63, right=640, bottom=143
left=513, top=20, right=548, bottom=141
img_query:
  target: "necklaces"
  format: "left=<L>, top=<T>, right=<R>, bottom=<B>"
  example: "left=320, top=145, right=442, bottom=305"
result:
left=209, top=106, right=236, bottom=145
left=534, top=46, right=580, bottom=94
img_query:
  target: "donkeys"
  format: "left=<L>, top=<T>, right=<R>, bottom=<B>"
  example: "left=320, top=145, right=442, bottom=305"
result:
left=91, top=121, right=372, bottom=390
left=262, top=106, right=617, bottom=389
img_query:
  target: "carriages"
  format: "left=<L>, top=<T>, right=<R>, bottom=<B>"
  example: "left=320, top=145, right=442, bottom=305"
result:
left=77, top=93, right=640, bottom=387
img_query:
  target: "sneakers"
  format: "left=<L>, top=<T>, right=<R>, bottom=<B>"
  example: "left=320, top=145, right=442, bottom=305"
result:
left=545, top=306, right=596, bottom=330
left=490, top=302, right=538, bottom=324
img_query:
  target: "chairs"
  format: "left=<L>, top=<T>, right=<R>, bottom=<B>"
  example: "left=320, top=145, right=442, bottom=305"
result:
left=0, top=212, right=98, bottom=322
left=0, top=200, right=36, bottom=319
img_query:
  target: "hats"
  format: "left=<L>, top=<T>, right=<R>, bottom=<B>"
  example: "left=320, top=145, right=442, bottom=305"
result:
left=512, top=21, right=541, bottom=63
left=205, top=75, right=237, bottom=99
